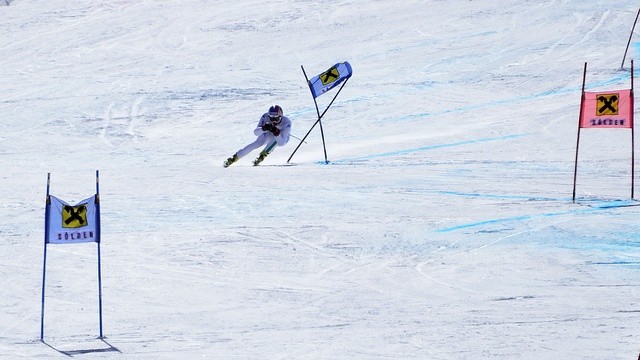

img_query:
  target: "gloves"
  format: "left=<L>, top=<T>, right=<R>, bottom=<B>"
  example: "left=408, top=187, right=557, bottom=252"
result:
left=262, top=124, right=271, bottom=131
left=269, top=125, right=280, bottom=136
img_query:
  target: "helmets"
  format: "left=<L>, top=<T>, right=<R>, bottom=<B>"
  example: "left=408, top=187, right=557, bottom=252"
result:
left=268, top=106, right=283, bottom=126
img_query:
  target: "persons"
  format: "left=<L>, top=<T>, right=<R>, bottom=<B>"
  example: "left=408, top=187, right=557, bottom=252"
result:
left=221, top=105, right=291, bottom=169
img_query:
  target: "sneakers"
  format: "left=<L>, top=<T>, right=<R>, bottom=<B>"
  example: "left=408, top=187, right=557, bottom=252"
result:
left=255, top=156, right=263, bottom=165
left=224, top=156, right=235, bottom=166
